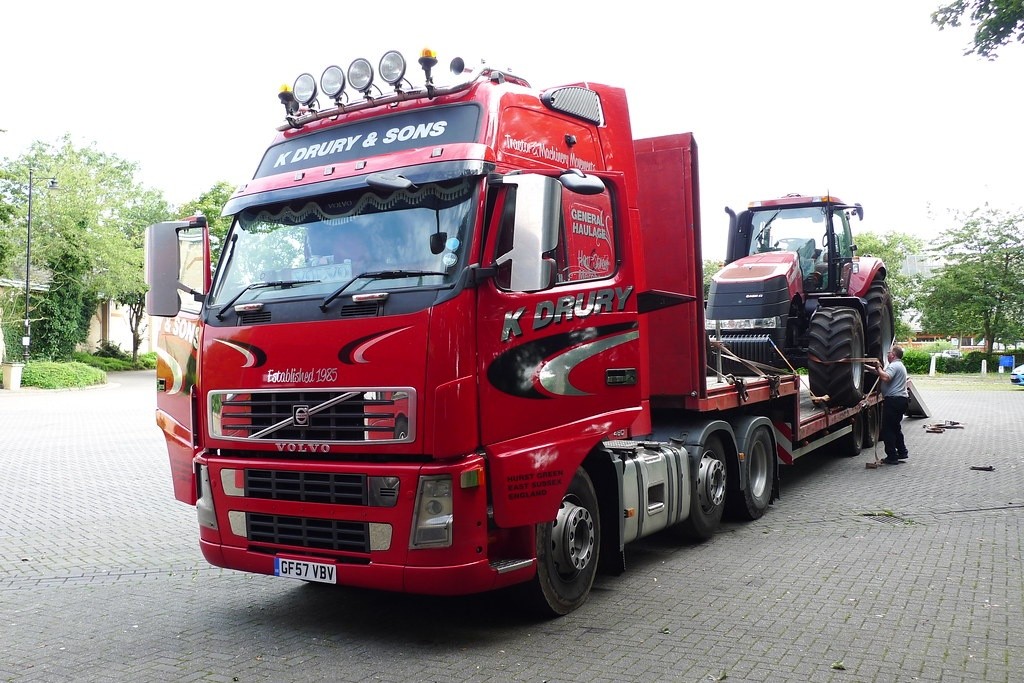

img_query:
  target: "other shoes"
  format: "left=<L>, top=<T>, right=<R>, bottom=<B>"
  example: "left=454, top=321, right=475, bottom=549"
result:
left=881, top=457, right=898, bottom=464
left=896, top=454, right=908, bottom=459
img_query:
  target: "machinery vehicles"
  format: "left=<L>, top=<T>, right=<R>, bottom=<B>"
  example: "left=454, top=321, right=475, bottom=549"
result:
left=704, top=187, right=894, bottom=407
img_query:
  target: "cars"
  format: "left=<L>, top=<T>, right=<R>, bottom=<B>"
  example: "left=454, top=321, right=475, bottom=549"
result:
left=1010, top=364, right=1024, bottom=387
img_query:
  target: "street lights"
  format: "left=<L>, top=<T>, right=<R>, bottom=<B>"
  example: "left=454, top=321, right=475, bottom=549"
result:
left=22, top=167, right=64, bottom=364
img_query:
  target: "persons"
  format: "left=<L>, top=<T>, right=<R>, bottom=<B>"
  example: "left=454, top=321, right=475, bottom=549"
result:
left=864, top=347, right=909, bottom=465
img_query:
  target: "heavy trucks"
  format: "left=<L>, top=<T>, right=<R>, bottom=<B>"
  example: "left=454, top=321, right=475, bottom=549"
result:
left=139, top=44, right=931, bottom=622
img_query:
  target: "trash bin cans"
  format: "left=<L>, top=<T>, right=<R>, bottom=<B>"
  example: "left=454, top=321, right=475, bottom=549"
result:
left=1, top=359, right=25, bottom=391
